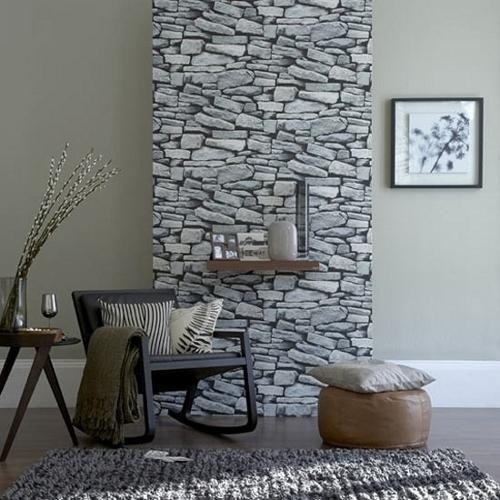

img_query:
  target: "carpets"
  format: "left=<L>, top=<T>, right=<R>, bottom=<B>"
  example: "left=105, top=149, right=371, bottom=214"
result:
left=0, top=446, right=499, bottom=499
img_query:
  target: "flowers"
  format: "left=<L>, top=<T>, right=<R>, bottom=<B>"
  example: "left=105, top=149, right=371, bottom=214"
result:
left=14, top=141, right=124, bottom=277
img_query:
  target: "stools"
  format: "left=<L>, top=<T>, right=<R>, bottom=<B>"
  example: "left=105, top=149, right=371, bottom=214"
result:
left=317, top=386, right=432, bottom=450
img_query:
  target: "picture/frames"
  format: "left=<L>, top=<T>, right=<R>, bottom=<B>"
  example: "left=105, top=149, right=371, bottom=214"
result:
left=391, top=97, right=483, bottom=188
left=210, top=232, right=239, bottom=261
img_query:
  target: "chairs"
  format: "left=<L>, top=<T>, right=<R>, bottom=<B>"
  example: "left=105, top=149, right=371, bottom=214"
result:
left=71, top=289, right=258, bottom=445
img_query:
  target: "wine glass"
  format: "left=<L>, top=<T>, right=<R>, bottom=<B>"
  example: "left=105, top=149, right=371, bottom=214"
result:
left=42, top=293, right=56, bottom=330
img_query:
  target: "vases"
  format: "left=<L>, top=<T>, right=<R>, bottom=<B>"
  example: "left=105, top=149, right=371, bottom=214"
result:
left=0, top=276, right=27, bottom=331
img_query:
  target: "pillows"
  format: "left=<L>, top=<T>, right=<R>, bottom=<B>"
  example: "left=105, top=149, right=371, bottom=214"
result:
left=308, top=358, right=436, bottom=394
left=99, top=297, right=176, bottom=355
left=169, top=298, right=224, bottom=353
